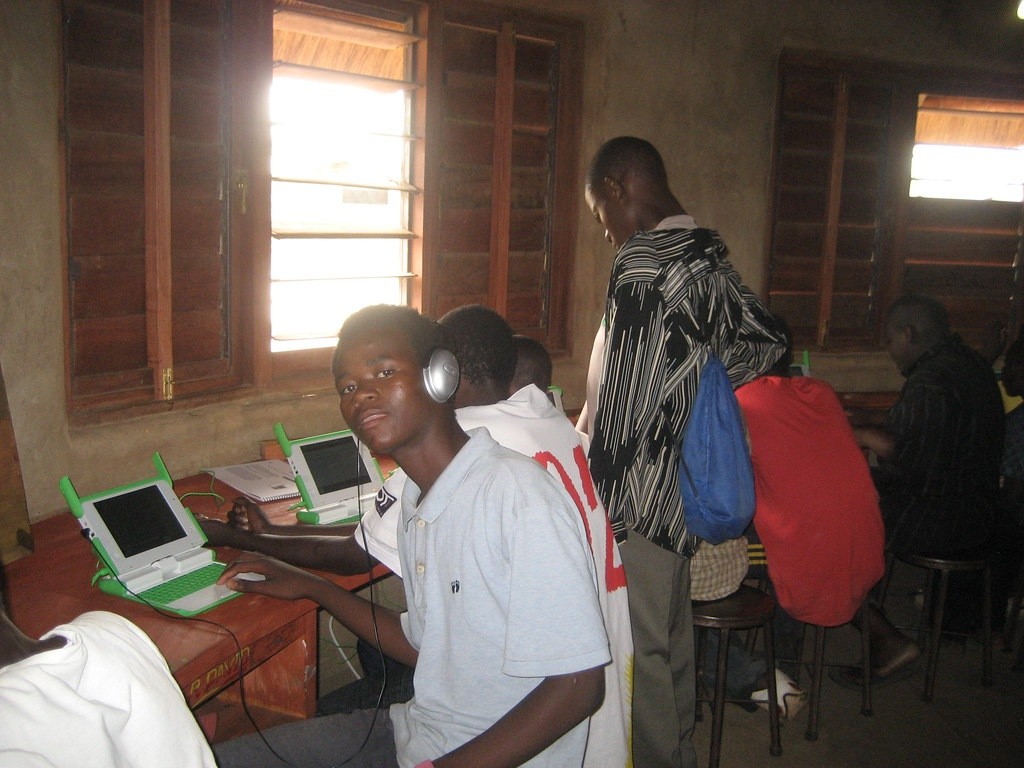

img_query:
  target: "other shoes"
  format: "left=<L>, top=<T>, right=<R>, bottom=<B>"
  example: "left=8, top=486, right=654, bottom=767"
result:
left=751, top=667, right=808, bottom=721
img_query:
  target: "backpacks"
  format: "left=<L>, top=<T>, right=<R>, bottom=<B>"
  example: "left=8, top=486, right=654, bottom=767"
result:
left=662, top=256, right=756, bottom=544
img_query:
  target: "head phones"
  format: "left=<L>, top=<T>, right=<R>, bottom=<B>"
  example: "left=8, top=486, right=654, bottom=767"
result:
left=419, top=323, right=460, bottom=404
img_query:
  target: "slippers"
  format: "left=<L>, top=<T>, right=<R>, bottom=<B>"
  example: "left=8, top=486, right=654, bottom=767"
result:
left=913, top=587, right=977, bottom=642
left=840, top=661, right=913, bottom=690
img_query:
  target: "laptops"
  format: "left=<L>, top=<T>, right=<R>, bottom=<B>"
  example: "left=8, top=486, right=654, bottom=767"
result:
left=545, top=387, right=568, bottom=418
left=788, top=349, right=811, bottom=380
left=269, top=422, right=389, bottom=528
left=59, top=452, right=267, bottom=616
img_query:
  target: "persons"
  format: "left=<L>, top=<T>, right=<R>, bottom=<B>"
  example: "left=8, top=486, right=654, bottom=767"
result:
left=0, top=560, right=220, bottom=767
left=690, top=535, right=811, bottom=721
left=200, top=302, right=635, bottom=768
left=214, top=306, right=613, bottom=768
left=576, top=137, right=791, bottom=768
left=839, top=294, right=1024, bottom=690
left=733, top=317, right=887, bottom=676
left=508, top=335, right=554, bottom=396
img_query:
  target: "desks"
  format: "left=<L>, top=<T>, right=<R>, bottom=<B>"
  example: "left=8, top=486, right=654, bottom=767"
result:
left=833, top=389, right=899, bottom=418
left=1, top=447, right=400, bottom=747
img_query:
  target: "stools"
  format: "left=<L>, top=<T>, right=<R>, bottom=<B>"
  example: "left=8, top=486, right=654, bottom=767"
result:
left=688, top=584, right=782, bottom=767
left=741, top=572, right=874, bottom=742
left=875, top=548, right=1001, bottom=702
